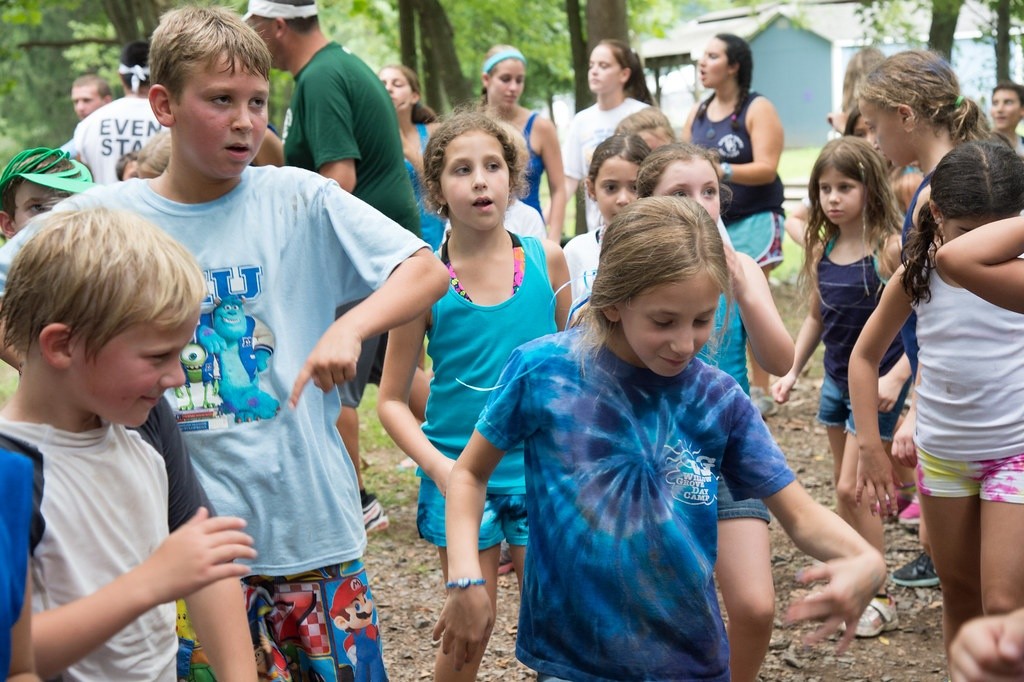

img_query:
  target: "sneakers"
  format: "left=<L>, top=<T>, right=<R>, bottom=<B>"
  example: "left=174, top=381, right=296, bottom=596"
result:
left=362, top=492, right=392, bottom=535
left=842, top=597, right=898, bottom=638
left=887, top=553, right=938, bottom=585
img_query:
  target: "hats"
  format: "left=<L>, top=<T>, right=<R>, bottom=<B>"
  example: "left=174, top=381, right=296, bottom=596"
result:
left=0, top=147, right=95, bottom=192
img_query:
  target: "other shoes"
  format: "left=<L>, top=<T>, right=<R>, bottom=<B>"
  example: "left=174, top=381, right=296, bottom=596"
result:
left=899, top=497, right=921, bottom=527
left=875, top=489, right=910, bottom=518
left=498, top=539, right=515, bottom=575
left=400, top=458, right=417, bottom=468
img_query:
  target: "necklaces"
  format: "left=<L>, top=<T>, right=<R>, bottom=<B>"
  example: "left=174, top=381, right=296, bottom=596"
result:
left=706, top=128, right=715, bottom=138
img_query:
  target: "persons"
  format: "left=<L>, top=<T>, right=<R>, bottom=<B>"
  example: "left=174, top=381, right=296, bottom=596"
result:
left=682, top=34, right=785, bottom=417
left=241, top=0, right=432, bottom=535
left=0, top=7, right=449, bottom=682
left=541, top=39, right=649, bottom=231
left=635, top=143, right=793, bottom=681
left=768, top=136, right=915, bottom=635
left=562, top=108, right=677, bottom=319
left=481, top=44, right=566, bottom=244
left=380, top=65, right=447, bottom=374
left=440, top=123, right=546, bottom=239
left=825, top=45, right=1024, bottom=682
left=375, top=110, right=571, bottom=682
left=434, top=199, right=888, bottom=682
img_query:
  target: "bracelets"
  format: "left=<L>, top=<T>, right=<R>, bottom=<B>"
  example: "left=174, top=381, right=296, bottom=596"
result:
left=448, top=578, right=485, bottom=589
left=721, top=163, right=730, bottom=183
